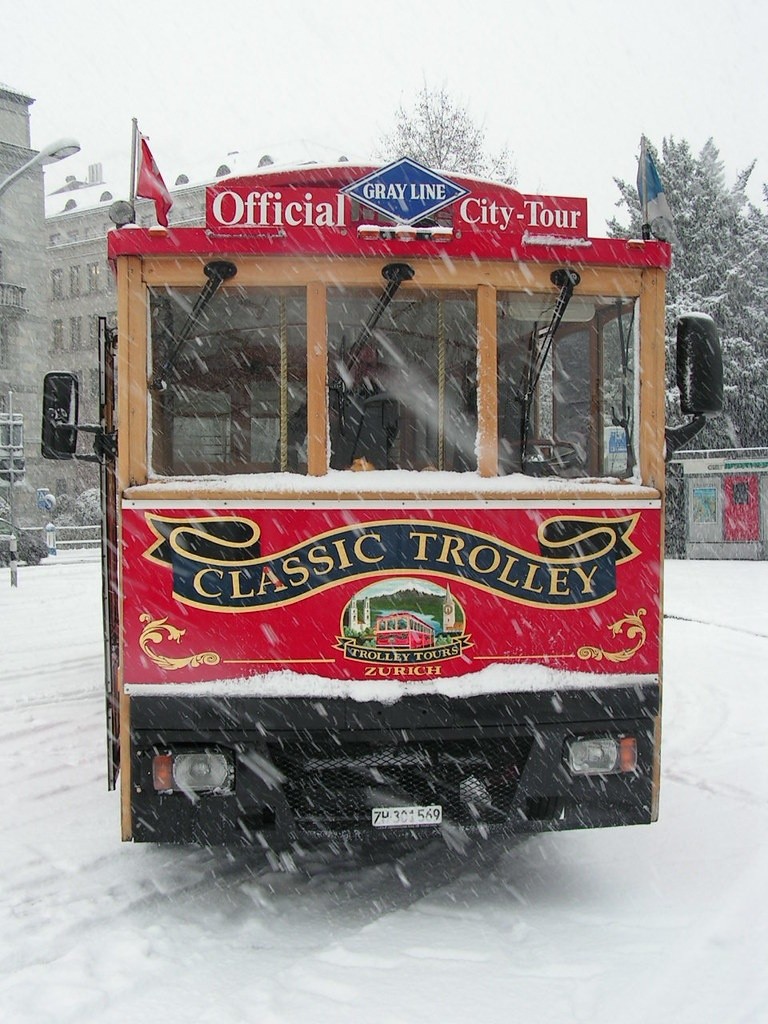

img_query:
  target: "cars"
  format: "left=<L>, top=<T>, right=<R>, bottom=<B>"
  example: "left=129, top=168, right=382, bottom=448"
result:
left=0, top=518, right=49, bottom=566
left=559, top=415, right=631, bottom=474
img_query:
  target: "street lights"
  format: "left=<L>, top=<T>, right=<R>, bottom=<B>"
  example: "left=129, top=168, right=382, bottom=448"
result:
left=0, top=137, right=82, bottom=198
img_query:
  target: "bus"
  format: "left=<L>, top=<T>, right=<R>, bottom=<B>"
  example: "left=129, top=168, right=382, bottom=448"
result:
left=40, top=157, right=724, bottom=846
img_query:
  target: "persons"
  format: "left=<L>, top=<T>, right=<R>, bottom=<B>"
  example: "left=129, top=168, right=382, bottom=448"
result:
left=272, top=340, right=400, bottom=474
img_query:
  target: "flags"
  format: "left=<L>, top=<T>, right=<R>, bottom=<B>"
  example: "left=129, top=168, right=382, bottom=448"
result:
left=637, top=146, right=676, bottom=241
left=135, top=129, right=173, bottom=227
